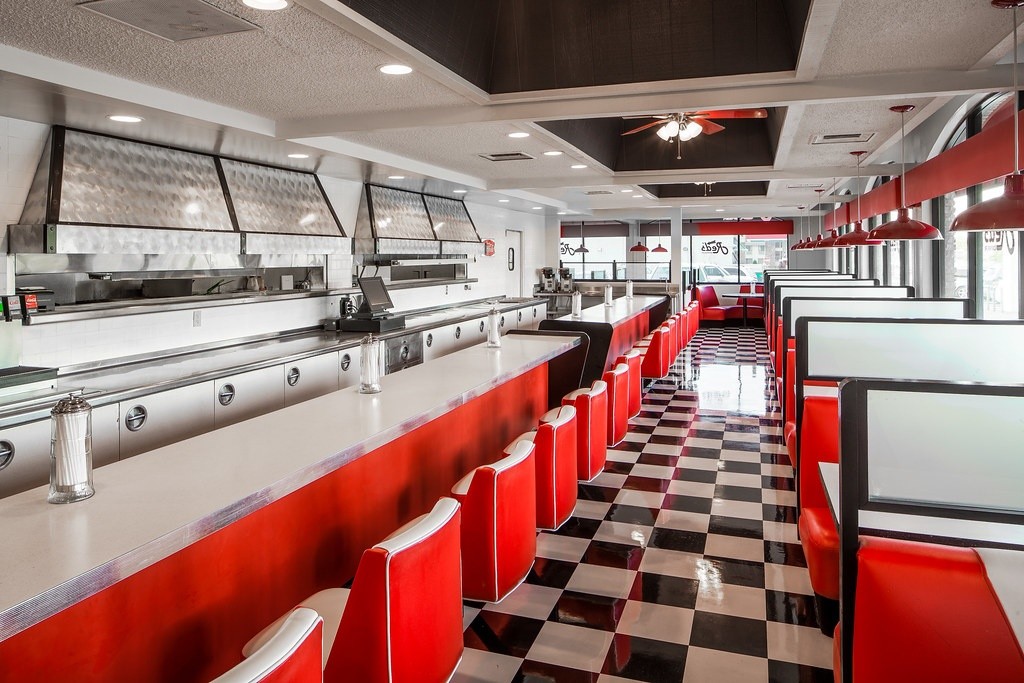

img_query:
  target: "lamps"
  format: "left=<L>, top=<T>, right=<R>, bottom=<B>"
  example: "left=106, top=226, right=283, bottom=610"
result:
left=796, top=188, right=813, bottom=250
left=816, top=177, right=851, bottom=249
left=656, top=113, right=704, bottom=160
left=629, top=219, right=650, bottom=251
left=949, top=0, right=1024, bottom=234
left=575, top=220, right=589, bottom=252
left=791, top=206, right=806, bottom=251
left=651, top=220, right=668, bottom=253
left=833, top=150, right=885, bottom=247
left=866, top=104, right=944, bottom=242
left=804, top=189, right=825, bottom=248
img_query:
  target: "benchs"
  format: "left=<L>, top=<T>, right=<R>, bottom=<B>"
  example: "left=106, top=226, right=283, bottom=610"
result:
left=761, top=266, right=1024, bottom=683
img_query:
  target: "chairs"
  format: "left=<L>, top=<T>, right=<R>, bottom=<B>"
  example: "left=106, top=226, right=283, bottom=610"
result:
left=213, top=299, right=701, bottom=683
left=690, top=285, right=737, bottom=325
left=732, top=285, right=765, bottom=328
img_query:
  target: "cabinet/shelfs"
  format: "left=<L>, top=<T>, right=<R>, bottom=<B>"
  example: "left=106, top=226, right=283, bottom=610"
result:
left=0, top=293, right=561, bottom=506
left=532, top=283, right=573, bottom=319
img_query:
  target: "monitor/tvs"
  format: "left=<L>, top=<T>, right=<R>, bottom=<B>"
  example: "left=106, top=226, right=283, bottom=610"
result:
left=358, top=276, right=394, bottom=311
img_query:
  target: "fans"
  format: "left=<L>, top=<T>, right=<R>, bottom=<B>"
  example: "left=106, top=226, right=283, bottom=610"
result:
left=618, top=110, right=770, bottom=136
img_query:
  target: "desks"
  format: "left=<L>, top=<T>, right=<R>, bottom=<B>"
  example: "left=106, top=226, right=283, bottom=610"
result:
left=722, top=292, right=765, bottom=329
left=537, top=291, right=672, bottom=412
left=0, top=329, right=595, bottom=682
